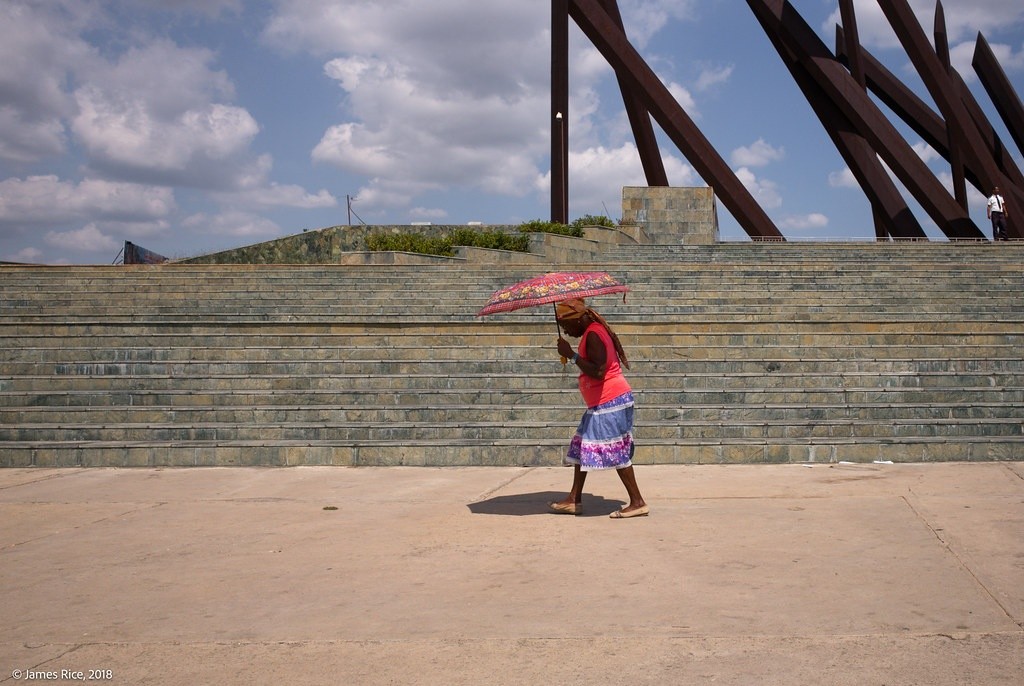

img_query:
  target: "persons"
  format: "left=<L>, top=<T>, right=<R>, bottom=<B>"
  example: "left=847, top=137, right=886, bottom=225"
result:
left=987, top=186, right=1010, bottom=241
left=546, top=298, right=649, bottom=518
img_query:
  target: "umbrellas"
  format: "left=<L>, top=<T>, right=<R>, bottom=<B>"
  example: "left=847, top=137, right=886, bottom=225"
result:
left=475, top=271, right=634, bottom=367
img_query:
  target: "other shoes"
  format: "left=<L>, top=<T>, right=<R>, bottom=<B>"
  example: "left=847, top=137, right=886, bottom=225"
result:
left=610, top=503, right=651, bottom=518
left=546, top=499, right=583, bottom=516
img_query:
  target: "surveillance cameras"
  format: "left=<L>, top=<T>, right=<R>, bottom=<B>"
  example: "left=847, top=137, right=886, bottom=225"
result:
left=556, top=114, right=563, bottom=121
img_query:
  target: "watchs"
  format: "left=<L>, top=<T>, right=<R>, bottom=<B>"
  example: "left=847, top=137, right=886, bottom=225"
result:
left=570, top=353, right=579, bottom=365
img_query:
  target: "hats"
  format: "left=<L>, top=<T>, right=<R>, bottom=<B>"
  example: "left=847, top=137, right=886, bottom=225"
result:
left=555, top=299, right=586, bottom=319
